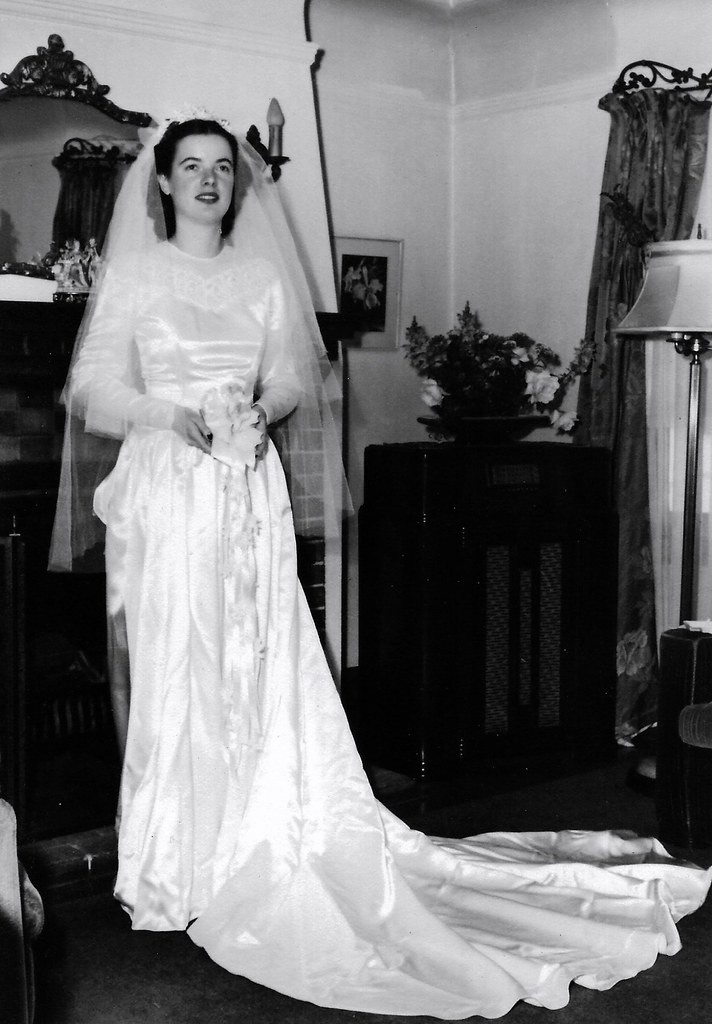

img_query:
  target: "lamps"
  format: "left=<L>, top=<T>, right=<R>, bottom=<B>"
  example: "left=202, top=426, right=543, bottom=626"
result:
left=608, top=240, right=712, bottom=633
left=248, top=96, right=289, bottom=182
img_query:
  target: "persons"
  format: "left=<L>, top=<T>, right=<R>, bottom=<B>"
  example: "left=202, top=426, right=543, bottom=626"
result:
left=47, top=101, right=331, bottom=931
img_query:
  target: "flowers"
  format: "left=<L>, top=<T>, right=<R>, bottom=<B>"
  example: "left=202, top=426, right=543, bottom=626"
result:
left=401, top=298, right=596, bottom=440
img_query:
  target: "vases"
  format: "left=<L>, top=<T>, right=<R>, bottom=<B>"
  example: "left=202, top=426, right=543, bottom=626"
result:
left=460, top=416, right=544, bottom=444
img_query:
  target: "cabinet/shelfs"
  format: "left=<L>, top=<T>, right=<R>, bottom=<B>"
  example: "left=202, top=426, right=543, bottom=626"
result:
left=361, top=439, right=621, bottom=780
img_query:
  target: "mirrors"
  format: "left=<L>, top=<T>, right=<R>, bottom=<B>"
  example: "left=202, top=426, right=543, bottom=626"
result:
left=0, top=35, right=153, bottom=280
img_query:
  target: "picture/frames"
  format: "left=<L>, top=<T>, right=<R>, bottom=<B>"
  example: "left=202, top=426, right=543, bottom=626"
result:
left=328, top=235, right=404, bottom=352
